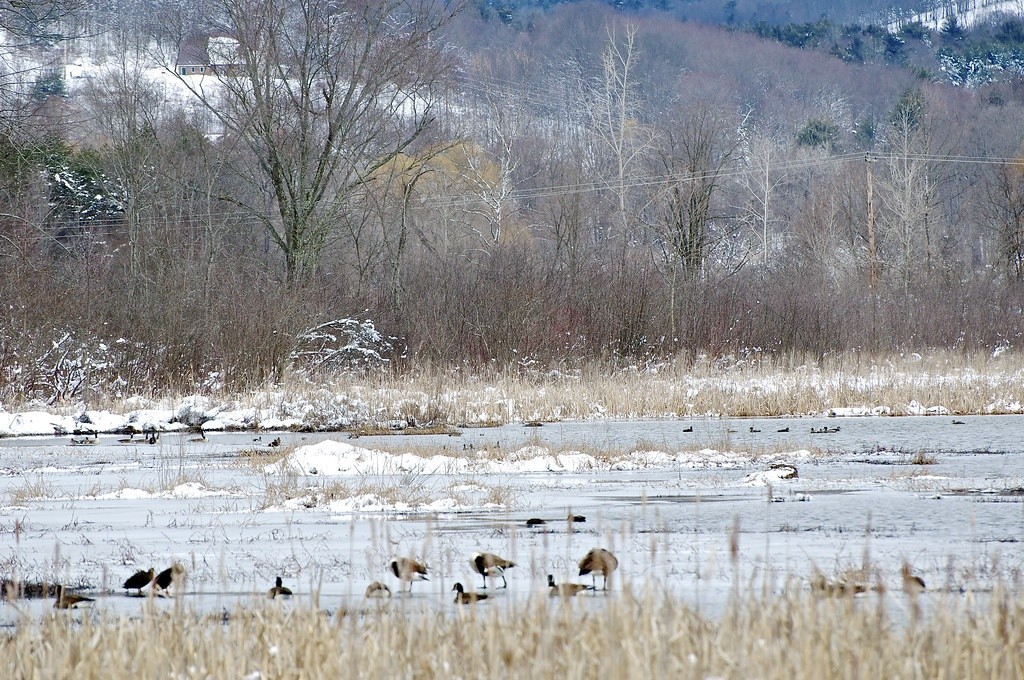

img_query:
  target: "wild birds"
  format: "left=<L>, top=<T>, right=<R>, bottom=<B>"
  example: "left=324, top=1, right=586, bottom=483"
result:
left=0, top=415, right=971, bottom=609
